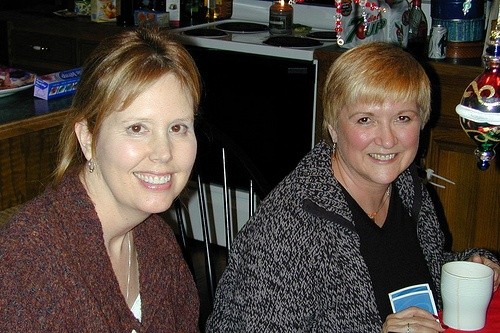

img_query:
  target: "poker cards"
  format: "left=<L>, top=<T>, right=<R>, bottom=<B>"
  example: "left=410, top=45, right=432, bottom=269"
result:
left=388, top=283, right=441, bottom=325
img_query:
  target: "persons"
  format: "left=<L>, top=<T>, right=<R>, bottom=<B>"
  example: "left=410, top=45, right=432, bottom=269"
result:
left=206, top=41, right=500, bottom=333
left=0, top=27, right=201, bottom=333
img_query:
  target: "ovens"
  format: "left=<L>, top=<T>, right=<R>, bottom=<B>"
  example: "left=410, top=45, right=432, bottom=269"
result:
left=169, top=32, right=318, bottom=199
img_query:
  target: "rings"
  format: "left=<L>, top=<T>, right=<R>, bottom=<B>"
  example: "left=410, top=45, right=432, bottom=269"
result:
left=407, top=323, right=410, bottom=333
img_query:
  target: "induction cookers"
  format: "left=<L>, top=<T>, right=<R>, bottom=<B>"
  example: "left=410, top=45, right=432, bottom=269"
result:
left=173, top=18, right=339, bottom=52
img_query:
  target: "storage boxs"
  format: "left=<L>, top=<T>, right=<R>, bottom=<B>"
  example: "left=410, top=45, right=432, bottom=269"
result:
left=133, top=8, right=171, bottom=28
left=90, top=0, right=117, bottom=22
left=32, top=66, right=85, bottom=101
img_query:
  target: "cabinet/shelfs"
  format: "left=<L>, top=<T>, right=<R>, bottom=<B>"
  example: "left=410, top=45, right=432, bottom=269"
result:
left=0, top=13, right=115, bottom=72
left=317, top=62, right=499, bottom=256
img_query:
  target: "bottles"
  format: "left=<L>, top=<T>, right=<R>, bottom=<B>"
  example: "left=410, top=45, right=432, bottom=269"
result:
left=119, top=0, right=231, bottom=30
left=269, top=0, right=293, bottom=35
left=407, top=0, right=428, bottom=62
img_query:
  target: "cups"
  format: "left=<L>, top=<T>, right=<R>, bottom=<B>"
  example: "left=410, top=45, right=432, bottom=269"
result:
left=440, top=261, right=494, bottom=331
left=75, top=1, right=90, bottom=16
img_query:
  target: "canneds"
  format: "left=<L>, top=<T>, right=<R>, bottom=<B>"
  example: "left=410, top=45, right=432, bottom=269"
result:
left=428, top=25, right=448, bottom=59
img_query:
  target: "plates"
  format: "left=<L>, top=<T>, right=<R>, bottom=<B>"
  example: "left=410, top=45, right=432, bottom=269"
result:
left=0, top=67, right=37, bottom=98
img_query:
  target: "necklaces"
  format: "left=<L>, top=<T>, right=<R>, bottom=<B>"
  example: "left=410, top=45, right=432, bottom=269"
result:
left=125, top=231, right=132, bottom=306
left=334, top=153, right=390, bottom=223
